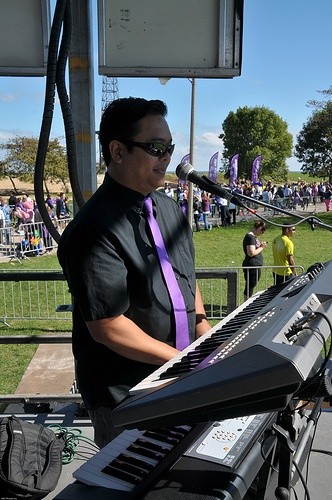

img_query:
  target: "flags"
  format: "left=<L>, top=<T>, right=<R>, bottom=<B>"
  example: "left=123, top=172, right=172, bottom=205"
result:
left=251, top=156, right=261, bottom=182
left=230, top=154, right=239, bottom=185
left=178, top=154, right=190, bottom=185
left=209, top=152, right=218, bottom=182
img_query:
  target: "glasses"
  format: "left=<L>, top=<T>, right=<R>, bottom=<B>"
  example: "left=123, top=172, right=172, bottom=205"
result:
left=118, top=139, right=175, bottom=157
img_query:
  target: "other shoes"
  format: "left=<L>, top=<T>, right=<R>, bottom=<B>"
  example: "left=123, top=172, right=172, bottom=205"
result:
left=15, top=230, right=20, bottom=234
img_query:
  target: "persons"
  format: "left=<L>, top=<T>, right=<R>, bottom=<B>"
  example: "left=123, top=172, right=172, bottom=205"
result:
left=218, top=198, right=232, bottom=227
left=0, top=191, right=72, bottom=257
left=242, top=221, right=268, bottom=301
left=272, top=222, right=297, bottom=284
left=200, top=193, right=212, bottom=230
left=57, top=97, right=213, bottom=449
left=229, top=202, right=236, bottom=225
left=193, top=198, right=200, bottom=232
left=164, top=182, right=220, bottom=221
left=231, top=178, right=332, bottom=215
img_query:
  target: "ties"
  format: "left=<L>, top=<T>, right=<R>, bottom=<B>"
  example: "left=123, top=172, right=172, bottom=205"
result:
left=142, top=197, right=189, bottom=351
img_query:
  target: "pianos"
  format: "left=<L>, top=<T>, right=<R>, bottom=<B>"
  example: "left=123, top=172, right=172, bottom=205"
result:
left=51, top=260, right=332, bottom=500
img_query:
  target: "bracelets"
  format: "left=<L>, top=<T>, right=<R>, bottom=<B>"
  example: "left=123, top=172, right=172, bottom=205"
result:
left=196, top=314, right=207, bottom=325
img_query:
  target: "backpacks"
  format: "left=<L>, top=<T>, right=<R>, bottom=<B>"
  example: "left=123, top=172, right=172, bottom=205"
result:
left=0, top=415, right=65, bottom=498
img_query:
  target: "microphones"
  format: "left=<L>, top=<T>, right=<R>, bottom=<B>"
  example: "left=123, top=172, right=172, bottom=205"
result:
left=176, top=162, right=245, bottom=207
